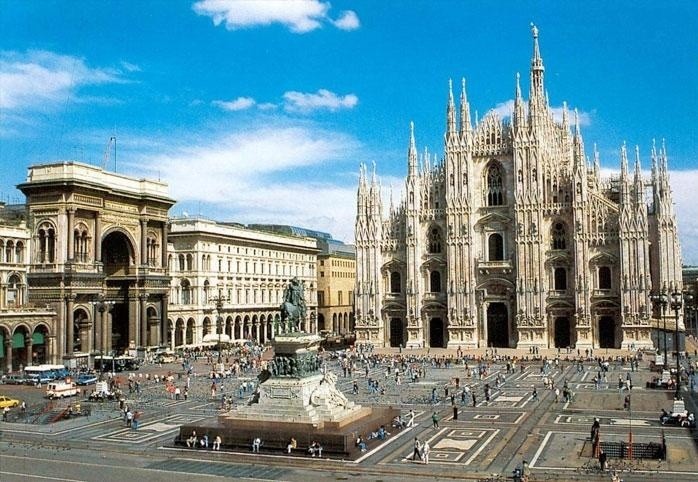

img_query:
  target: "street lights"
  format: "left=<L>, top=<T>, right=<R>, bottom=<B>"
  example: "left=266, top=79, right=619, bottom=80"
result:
left=649, top=282, right=694, bottom=418
left=208, top=289, right=232, bottom=374
left=89, top=294, right=115, bottom=396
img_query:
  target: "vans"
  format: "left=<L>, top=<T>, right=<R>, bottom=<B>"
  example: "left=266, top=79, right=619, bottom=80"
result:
left=24, top=364, right=97, bottom=399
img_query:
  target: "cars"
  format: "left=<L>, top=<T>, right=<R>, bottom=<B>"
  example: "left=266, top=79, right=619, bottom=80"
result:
left=2, top=375, right=26, bottom=385
left=0, top=395, right=19, bottom=409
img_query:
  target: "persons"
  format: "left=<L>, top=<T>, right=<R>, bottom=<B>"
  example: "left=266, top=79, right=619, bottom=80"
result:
left=512, top=463, right=521, bottom=481
left=308, top=439, right=316, bottom=453
left=421, top=441, right=430, bottom=465
left=286, top=275, right=306, bottom=306
left=250, top=436, right=261, bottom=453
left=311, top=441, right=323, bottom=457
left=186, top=430, right=198, bottom=448
left=413, top=437, right=422, bottom=461
left=598, top=449, right=607, bottom=470
left=356, top=434, right=367, bottom=452
left=2, top=341, right=698, bottom=431
left=378, top=425, right=386, bottom=441
left=286, top=437, right=297, bottom=454
left=199, top=434, right=209, bottom=448
left=212, top=435, right=222, bottom=451
left=521, top=459, right=531, bottom=481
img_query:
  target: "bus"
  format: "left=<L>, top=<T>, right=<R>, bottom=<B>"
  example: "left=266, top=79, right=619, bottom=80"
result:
left=94, top=355, right=139, bottom=372
